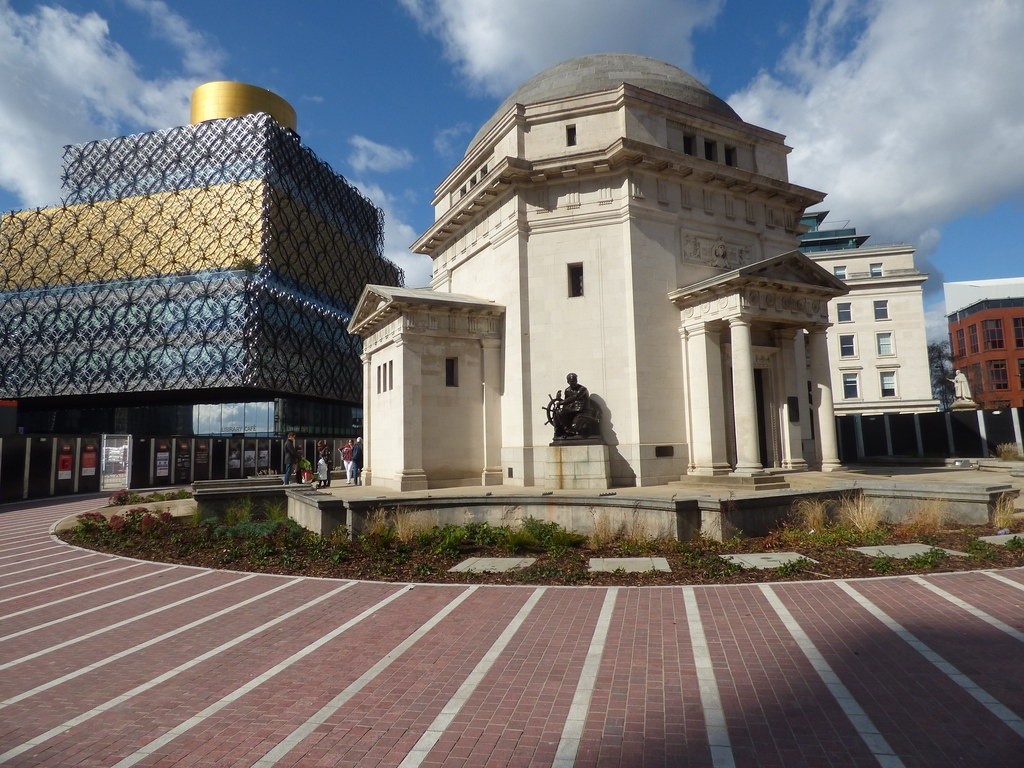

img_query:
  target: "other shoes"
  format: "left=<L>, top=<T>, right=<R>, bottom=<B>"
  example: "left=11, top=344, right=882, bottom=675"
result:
left=346, top=481, right=351, bottom=485
left=322, top=484, right=330, bottom=488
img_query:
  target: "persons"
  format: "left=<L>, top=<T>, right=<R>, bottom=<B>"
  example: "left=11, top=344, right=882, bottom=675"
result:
left=284, top=432, right=304, bottom=485
left=316, top=440, right=333, bottom=489
left=554, top=373, right=594, bottom=438
left=337, top=437, right=363, bottom=486
left=948, top=370, right=968, bottom=400
